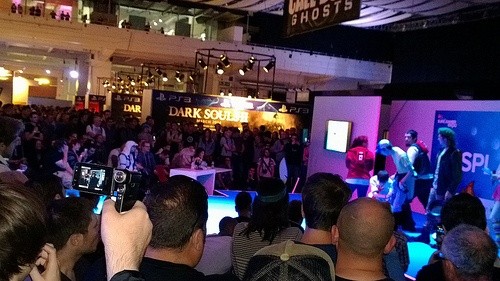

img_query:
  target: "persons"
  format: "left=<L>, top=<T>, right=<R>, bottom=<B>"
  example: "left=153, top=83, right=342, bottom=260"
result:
left=79, top=172, right=101, bottom=190
left=11, top=3, right=165, bottom=34
left=0, top=101, right=500, bottom=281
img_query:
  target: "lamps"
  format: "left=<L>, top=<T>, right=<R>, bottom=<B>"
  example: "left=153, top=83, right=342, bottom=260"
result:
left=263, top=63, right=273, bottom=72
left=145, top=70, right=154, bottom=79
left=246, top=57, right=255, bottom=71
left=220, top=55, right=232, bottom=69
left=175, top=71, right=180, bottom=77
left=154, top=70, right=162, bottom=78
left=144, top=78, right=152, bottom=86
left=176, top=74, right=185, bottom=82
left=239, top=66, right=247, bottom=76
left=189, top=72, right=198, bottom=81
left=198, top=59, right=207, bottom=70
left=216, top=62, right=224, bottom=75
left=163, top=72, right=168, bottom=81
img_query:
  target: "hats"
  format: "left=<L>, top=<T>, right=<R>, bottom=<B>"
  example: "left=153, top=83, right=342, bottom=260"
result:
left=376, top=139, right=391, bottom=154
left=244, top=239, right=335, bottom=281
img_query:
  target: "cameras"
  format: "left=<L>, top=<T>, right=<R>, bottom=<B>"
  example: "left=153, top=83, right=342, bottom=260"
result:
left=71, top=162, right=144, bottom=214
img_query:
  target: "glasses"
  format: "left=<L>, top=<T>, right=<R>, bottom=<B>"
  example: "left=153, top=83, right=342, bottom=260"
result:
left=433, top=251, right=460, bottom=269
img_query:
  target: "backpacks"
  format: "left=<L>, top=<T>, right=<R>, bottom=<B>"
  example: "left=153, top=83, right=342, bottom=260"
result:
left=411, top=143, right=430, bottom=175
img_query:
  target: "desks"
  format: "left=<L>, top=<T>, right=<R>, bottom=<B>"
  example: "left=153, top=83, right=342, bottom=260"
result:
left=169, top=167, right=232, bottom=196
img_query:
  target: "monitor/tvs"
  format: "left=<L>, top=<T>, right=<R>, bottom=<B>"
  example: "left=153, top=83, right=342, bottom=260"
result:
left=324, top=120, right=352, bottom=152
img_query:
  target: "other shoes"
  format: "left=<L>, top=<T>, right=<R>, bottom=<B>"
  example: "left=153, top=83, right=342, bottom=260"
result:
left=414, top=236, right=430, bottom=243
